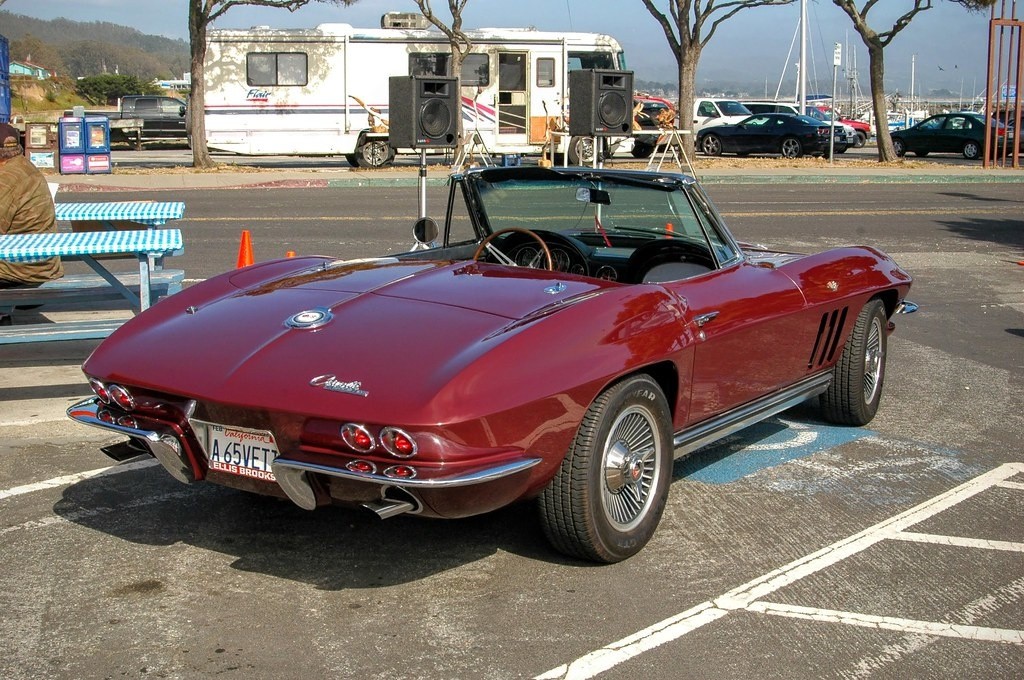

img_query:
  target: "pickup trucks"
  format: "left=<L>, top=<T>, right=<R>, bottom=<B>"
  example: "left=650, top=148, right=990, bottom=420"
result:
left=672, top=98, right=784, bottom=135
left=60, top=95, right=189, bottom=144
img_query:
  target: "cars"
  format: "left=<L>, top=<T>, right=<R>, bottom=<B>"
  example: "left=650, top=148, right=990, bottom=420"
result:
left=1004, top=114, right=1024, bottom=153
left=735, top=102, right=871, bottom=154
left=890, top=111, right=1021, bottom=160
left=695, top=112, right=847, bottom=159
left=632, top=96, right=677, bottom=159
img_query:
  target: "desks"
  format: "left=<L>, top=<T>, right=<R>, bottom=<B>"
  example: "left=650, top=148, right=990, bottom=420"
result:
left=550, top=130, right=698, bottom=180
left=54, top=201, right=185, bottom=271
left=0, top=228, right=184, bottom=311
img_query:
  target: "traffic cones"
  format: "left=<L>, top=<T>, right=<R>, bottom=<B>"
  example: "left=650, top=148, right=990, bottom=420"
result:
left=234, top=229, right=254, bottom=269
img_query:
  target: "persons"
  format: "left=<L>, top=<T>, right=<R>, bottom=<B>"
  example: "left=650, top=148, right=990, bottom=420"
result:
left=0, top=123, right=65, bottom=326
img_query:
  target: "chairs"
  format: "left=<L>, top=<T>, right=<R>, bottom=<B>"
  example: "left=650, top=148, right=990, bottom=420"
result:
left=698, top=106, right=707, bottom=116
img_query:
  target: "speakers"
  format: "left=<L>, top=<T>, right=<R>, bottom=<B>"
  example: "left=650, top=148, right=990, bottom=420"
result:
left=389, top=75, right=459, bottom=149
left=569, top=70, right=635, bottom=137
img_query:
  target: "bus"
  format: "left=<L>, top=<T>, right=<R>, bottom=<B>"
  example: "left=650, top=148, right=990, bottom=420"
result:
left=195, top=11, right=634, bottom=172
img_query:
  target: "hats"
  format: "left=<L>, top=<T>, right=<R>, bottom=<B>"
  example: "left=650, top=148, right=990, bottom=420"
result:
left=0, top=122, right=20, bottom=148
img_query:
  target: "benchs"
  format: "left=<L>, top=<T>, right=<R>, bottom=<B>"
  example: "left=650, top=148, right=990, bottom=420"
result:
left=0, top=267, right=187, bottom=345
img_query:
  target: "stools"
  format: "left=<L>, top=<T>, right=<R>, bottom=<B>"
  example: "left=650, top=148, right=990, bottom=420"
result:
left=501, top=152, right=521, bottom=167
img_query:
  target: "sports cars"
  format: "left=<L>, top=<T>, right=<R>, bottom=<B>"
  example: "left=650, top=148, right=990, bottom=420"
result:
left=65, top=162, right=887, bottom=562
